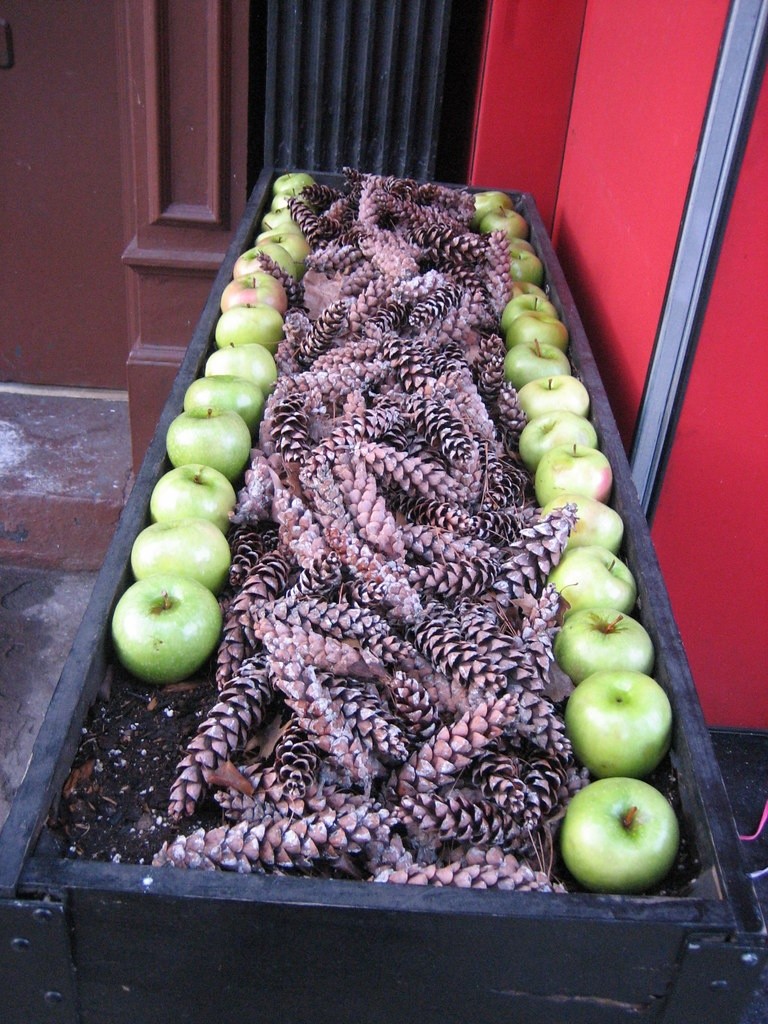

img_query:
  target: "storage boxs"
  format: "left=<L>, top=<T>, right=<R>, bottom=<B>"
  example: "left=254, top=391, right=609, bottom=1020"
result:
left=0, top=168, right=766, bottom=1024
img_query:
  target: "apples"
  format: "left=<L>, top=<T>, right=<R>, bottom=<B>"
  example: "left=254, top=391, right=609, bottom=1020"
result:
left=546, top=548, right=637, bottom=616
left=563, top=778, right=678, bottom=893
left=541, top=493, right=626, bottom=554
left=112, top=171, right=317, bottom=684
left=553, top=607, right=655, bottom=684
left=469, top=189, right=614, bottom=509
left=565, top=669, right=673, bottom=779
